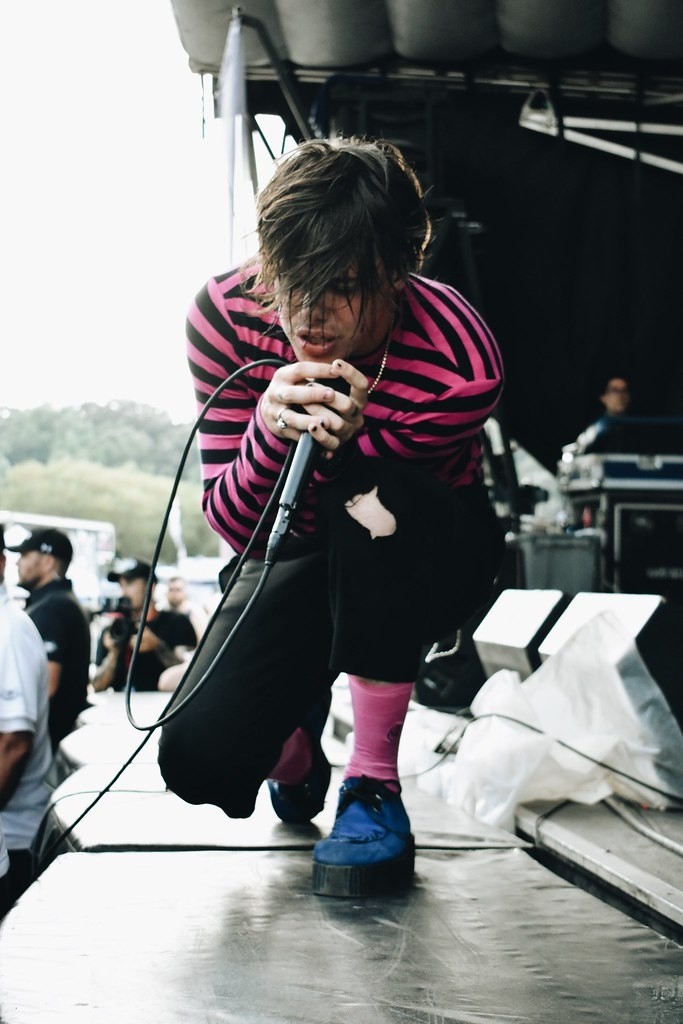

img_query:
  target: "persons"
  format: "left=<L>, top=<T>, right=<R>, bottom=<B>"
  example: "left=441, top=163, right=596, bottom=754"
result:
left=157, top=132, right=502, bottom=902
left=85, top=558, right=196, bottom=693
left=0, top=525, right=48, bottom=920
left=567, top=369, right=649, bottom=454
left=157, top=573, right=208, bottom=648
left=5, top=523, right=91, bottom=756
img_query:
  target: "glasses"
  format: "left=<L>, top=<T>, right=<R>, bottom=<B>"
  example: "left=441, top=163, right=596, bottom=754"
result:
left=608, top=386, right=629, bottom=395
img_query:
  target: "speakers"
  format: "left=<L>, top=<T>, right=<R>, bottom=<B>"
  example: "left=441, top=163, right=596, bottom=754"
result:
left=475, top=501, right=683, bottom=735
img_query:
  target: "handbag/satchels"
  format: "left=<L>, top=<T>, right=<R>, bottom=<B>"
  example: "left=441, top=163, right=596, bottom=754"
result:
left=414, top=626, right=488, bottom=707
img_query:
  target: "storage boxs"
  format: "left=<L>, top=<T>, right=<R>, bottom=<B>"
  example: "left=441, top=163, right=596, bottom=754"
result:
left=557, top=453, right=683, bottom=491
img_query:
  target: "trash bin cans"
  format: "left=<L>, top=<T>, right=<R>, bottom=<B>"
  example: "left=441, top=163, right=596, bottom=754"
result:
left=525, top=535, right=616, bottom=598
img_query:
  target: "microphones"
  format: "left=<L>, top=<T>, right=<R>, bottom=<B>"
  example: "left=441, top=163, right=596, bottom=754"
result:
left=264, top=375, right=350, bottom=566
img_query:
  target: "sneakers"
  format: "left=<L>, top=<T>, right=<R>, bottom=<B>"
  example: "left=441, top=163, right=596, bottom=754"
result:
left=313, top=774, right=417, bottom=897
left=268, top=687, right=332, bottom=822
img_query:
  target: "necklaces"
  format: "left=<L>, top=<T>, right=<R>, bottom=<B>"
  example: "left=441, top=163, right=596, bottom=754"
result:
left=291, top=291, right=399, bottom=398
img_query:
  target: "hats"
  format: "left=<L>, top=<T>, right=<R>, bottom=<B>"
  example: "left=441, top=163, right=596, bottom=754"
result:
left=5, top=529, right=73, bottom=560
left=107, top=559, right=157, bottom=582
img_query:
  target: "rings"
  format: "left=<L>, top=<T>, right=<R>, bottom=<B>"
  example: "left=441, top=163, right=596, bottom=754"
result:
left=276, top=406, right=289, bottom=429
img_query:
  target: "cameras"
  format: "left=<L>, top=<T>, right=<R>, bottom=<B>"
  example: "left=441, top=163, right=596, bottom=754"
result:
left=109, top=596, right=139, bottom=654
